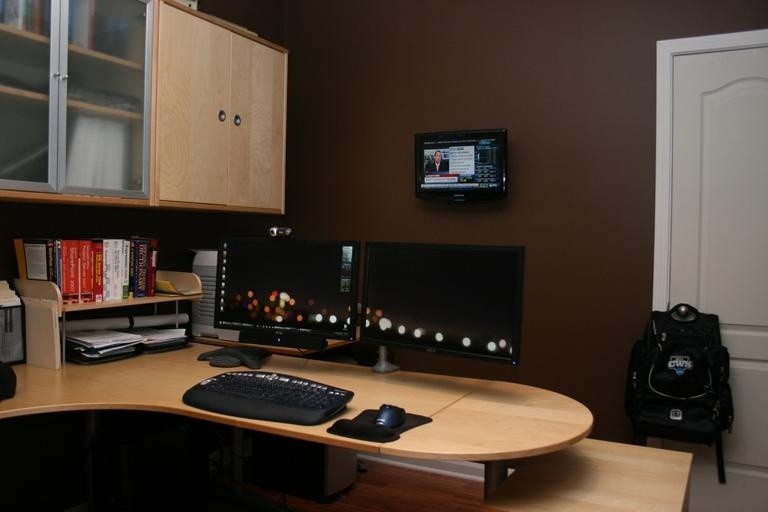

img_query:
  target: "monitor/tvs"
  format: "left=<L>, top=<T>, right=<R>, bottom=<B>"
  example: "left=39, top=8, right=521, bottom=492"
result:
left=414, top=129, right=509, bottom=211
left=214, top=235, right=357, bottom=350
left=359, top=240, right=524, bottom=368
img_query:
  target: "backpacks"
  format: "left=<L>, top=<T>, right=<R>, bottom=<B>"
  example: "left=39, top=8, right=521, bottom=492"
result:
left=624, top=301, right=735, bottom=447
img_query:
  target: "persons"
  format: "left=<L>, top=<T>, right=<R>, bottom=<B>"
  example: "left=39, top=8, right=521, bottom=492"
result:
left=428, top=150, right=448, bottom=172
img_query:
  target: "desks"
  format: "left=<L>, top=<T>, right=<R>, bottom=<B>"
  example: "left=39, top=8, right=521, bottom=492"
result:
left=0, top=330, right=696, bottom=511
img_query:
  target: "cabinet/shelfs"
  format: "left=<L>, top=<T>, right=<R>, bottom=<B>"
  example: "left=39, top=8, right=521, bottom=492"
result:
left=0, top=0, right=153, bottom=206
left=150, top=1, right=289, bottom=215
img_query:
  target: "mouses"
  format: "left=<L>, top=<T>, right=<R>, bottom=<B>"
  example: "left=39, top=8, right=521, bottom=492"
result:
left=375, top=402, right=406, bottom=429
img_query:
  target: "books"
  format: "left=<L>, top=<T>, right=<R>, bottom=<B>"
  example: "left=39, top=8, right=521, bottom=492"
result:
left=22, top=233, right=160, bottom=303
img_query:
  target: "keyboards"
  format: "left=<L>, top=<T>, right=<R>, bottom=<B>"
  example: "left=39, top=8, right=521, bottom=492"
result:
left=183, top=370, right=354, bottom=426
